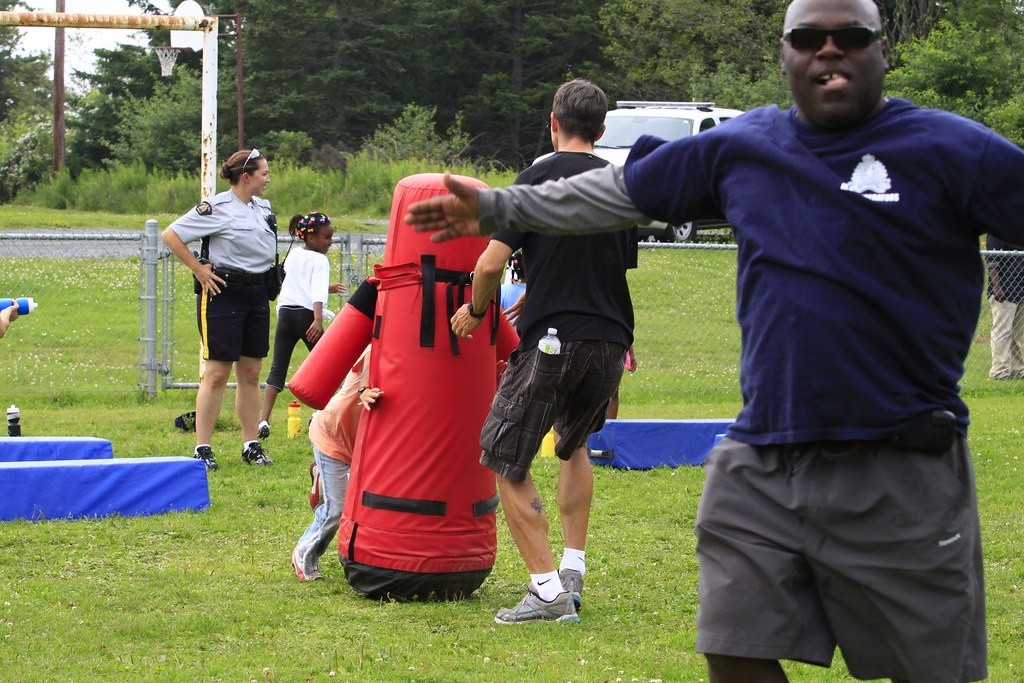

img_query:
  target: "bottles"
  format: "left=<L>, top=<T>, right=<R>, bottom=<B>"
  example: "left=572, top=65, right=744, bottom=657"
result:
left=538, top=327, right=561, bottom=355
left=287, top=399, right=301, bottom=437
left=7, top=404, right=21, bottom=437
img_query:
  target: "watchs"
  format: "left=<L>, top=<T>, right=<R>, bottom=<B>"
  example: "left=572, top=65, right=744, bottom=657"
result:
left=467, top=299, right=487, bottom=319
left=358, top=386, right=365, bottom=393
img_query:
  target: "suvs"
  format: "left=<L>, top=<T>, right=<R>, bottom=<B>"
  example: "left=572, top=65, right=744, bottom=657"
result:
left=533, top=99, right=750, bottom=248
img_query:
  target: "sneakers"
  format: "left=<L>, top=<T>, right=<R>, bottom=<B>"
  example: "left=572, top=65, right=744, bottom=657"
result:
left=291, top=557, right=321, bottom=579
left=241, top=441, right=271, bottom=465
left=529, top=568, right=584, bottom=608
left=194, top=446, right=217, bottom=470
left=310, top=463, right=323, bottom=510
left=495, top=583, right=580, bottom=624
left=257, top=425, right=269, bottom=438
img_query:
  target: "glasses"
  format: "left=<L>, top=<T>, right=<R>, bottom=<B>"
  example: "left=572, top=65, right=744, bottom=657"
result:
left=242, top=148, right=260, bottom=172
left=780, top=24, right=881, bottom=52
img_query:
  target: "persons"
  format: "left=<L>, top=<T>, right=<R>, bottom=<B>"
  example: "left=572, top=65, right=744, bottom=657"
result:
left=405, top=0, right=1024, bottom=683
left=500, top=261, right=637, bottom=418
left=291, top=343, right=384, bottom=581
left=257, top=212, right=347, bottom=440
left=160, top=149, right=276, bottom=469
left=450, top=79, right=639, bottom=628
left=0, top=300, right=19, bottom=337
left=984, top=234, right=1024, bottom=380
